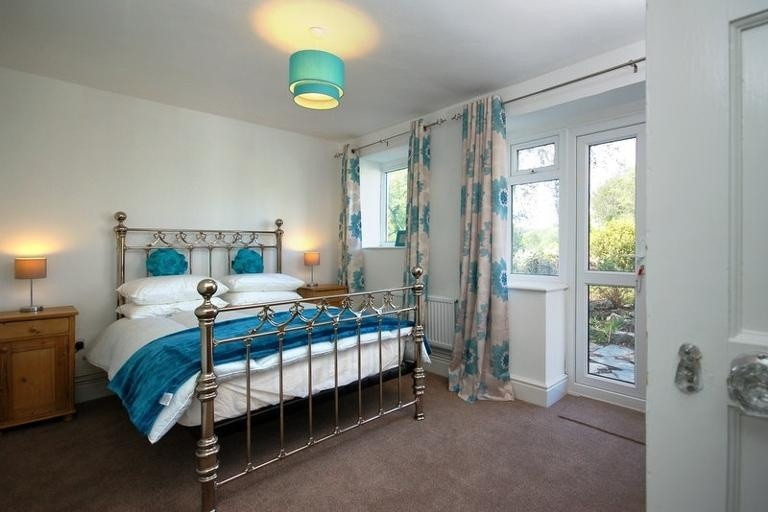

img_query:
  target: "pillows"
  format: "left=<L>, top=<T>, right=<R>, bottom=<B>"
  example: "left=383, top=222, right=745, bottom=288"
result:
left=116, top=272, right=229, bottom=305
left=225, top=292, right=303, bottom=308
left=221, top=271, right=305, bottom=293
left=114, top=297, right=230, bottom=320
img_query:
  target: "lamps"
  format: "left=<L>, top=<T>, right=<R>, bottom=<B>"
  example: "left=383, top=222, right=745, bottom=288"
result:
left=304, top=252, right=320, bottom=287
left=14, top=257, right=47, bottom=313
left=286, top=47, right=346, bottom=111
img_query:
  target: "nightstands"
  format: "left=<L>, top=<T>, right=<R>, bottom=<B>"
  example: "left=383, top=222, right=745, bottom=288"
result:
left=297, top=284, right=349, bottom=307
left=0, top=304, right=80, bottom=431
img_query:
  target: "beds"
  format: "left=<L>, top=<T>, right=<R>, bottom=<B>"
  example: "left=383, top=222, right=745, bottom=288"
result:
left=112, top=211, right=426, bottom=512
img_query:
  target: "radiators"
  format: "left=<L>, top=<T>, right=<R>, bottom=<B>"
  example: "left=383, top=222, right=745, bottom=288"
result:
left=385, top=289, right=458, bottom=352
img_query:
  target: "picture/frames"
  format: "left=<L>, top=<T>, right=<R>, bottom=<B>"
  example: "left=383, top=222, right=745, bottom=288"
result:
left=395, top=230, right=406, bottom=246
